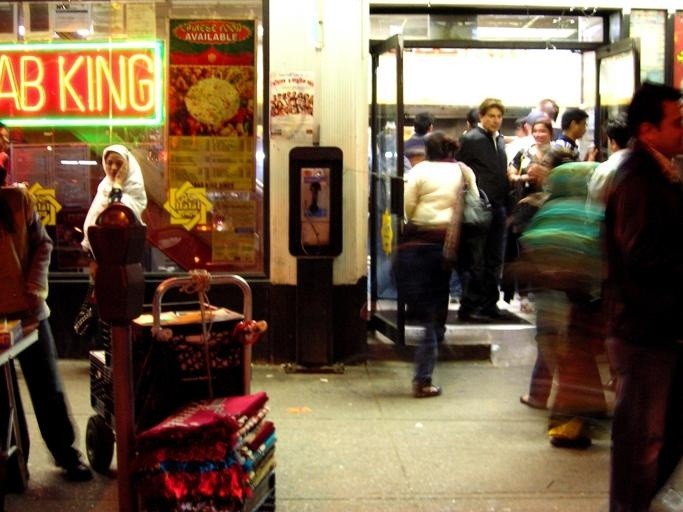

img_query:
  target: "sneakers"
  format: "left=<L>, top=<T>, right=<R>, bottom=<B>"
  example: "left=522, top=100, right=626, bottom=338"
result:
left=448, top=289, right=536, bottom=324
left=412, top=380, right=442, bottom=398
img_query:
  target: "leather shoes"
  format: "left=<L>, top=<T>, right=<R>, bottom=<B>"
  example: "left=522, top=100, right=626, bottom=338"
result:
left=0, top=466, right=30, bottom=494
left=62, top=459, right=94, bottom=483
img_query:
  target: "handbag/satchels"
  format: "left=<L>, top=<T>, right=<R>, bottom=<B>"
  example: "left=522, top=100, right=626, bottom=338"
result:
left=462, top=186, right=493, bottom=229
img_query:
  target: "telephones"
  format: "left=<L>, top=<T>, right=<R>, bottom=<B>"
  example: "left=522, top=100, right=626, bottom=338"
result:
left=299, top=167, right=330, bottom=246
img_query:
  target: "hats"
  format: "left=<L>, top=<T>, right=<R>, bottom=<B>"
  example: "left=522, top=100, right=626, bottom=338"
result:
left=526, top=110, right=548, bottom=127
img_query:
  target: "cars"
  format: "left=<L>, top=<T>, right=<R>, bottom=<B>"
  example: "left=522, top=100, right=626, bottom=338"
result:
left=1, top=142, right=263, bottom=275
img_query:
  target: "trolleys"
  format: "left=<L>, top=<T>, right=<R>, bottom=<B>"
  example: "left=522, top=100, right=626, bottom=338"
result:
left=82, top=272, right=253, bottom=472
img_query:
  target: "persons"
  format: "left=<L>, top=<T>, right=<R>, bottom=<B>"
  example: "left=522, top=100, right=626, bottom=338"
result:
left=372, top=98, right=635, bottom=443
left=78, top=142, right=149, bottom=270
left=0, top=122, right=13, bottom=189
left=593, top=78, right=683, bottom=511
left=0, top=183, right=95, bottom=489
